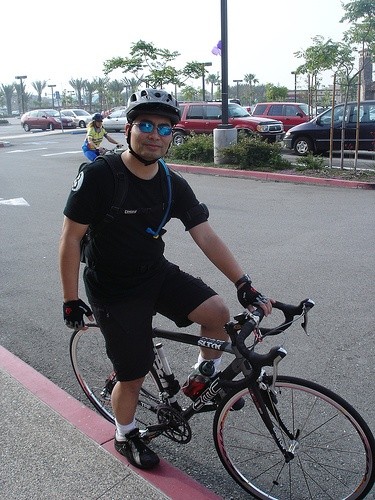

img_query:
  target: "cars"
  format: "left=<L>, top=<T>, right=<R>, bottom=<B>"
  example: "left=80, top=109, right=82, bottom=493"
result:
left=21, top=109, right=76, bottom=132
left=283, top=100, right=375, bottom=156
left=101, top=105, right=128, bottom=132
left=59, top=110, right=91, bottom=128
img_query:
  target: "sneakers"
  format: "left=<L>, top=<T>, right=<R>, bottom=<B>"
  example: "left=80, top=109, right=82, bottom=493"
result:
left=183, top=368, right=246, bottom=412
left=114, top=427, right=162, bottom=472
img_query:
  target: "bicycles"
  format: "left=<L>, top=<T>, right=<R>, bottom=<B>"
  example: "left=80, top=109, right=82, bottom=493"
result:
left=70, top=294, right=375, bottom=500
left=79, top=145, right=126, bottom=173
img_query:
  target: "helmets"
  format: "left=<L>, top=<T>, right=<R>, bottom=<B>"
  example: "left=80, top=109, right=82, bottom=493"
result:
left=92, top=113, right=103, bottom=120
left=125, top=89, right=181, bottom=124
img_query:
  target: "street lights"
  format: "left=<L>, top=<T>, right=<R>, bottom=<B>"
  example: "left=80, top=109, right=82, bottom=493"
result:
left=292, top=71, right=300, bottom=103
left=195, top=62, right=212, bottom=101
left=48, top=85, right=56, bottom=109
left=233, top=80, right=242, bottom=99
left=15, top=76, right=27, bottom=114
left=123, top=86, right=130, bottom=106
left=68, top=91, right=75, bottom=106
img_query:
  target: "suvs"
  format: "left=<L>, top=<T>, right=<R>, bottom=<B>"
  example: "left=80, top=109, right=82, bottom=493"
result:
left=251, top=103, right=318, bottom=133
left=172, top=101, right=285, bottom=149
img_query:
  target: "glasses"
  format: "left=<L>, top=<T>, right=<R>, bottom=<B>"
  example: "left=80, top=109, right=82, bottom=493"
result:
left=97, top=121, right=103, bottom=122
left=132, top=121, right=172, bottom=136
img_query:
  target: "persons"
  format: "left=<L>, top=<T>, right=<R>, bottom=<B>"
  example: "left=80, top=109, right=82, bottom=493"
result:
left=60, top=88, right=274, bottom=472
left=83, top=114, right=122, bottom=162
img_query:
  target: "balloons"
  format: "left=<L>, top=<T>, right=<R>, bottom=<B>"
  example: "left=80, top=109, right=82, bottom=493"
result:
left=212, top=40, right=221, bottom=56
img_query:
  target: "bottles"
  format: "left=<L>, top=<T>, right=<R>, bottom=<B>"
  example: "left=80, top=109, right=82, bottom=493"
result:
left=181, top=359, right=215, bottom=396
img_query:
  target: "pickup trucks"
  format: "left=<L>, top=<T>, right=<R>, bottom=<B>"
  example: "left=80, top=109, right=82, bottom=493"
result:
left=211, top=98, right=253, bottom=116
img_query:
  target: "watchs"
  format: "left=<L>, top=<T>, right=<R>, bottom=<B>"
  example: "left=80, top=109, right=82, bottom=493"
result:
left=234, top=274, right=251, bottom=288
